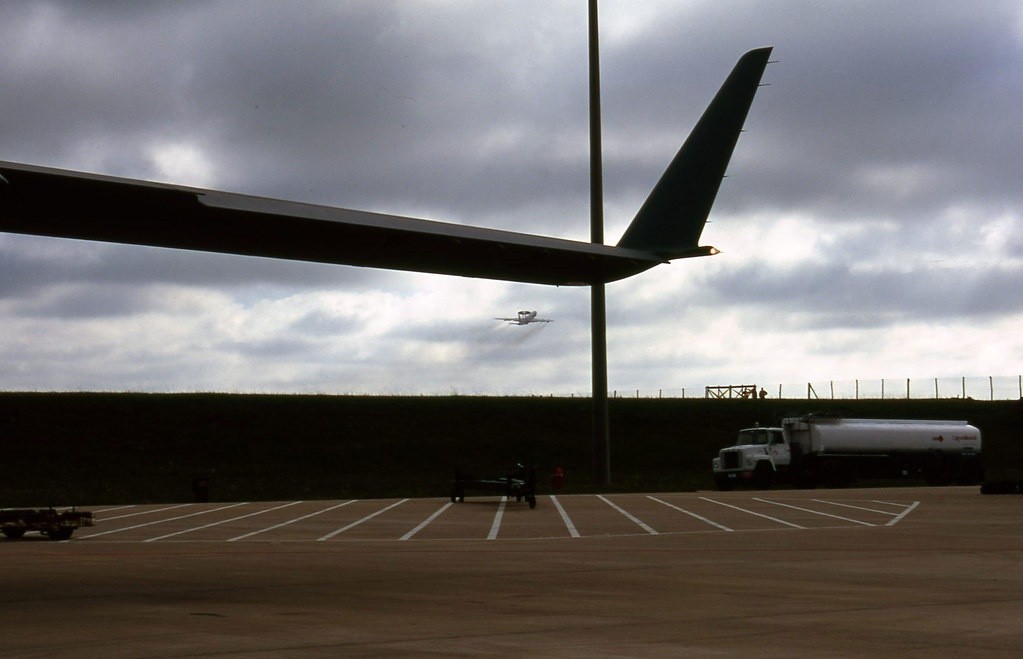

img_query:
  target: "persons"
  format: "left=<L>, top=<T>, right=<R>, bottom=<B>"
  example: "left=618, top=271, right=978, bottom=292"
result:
left=504, top=461, right=538, bottom=508
left=448, top=462, right=467, bottom=503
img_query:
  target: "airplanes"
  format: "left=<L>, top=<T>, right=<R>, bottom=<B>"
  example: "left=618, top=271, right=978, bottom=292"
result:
left=495, top=310, right=554, bottom=325
left=0, top=46, right=774, bottom=288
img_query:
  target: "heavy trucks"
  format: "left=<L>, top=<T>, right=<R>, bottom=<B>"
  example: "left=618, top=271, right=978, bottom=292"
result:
left=712, top=409, right=985, bottom=490
